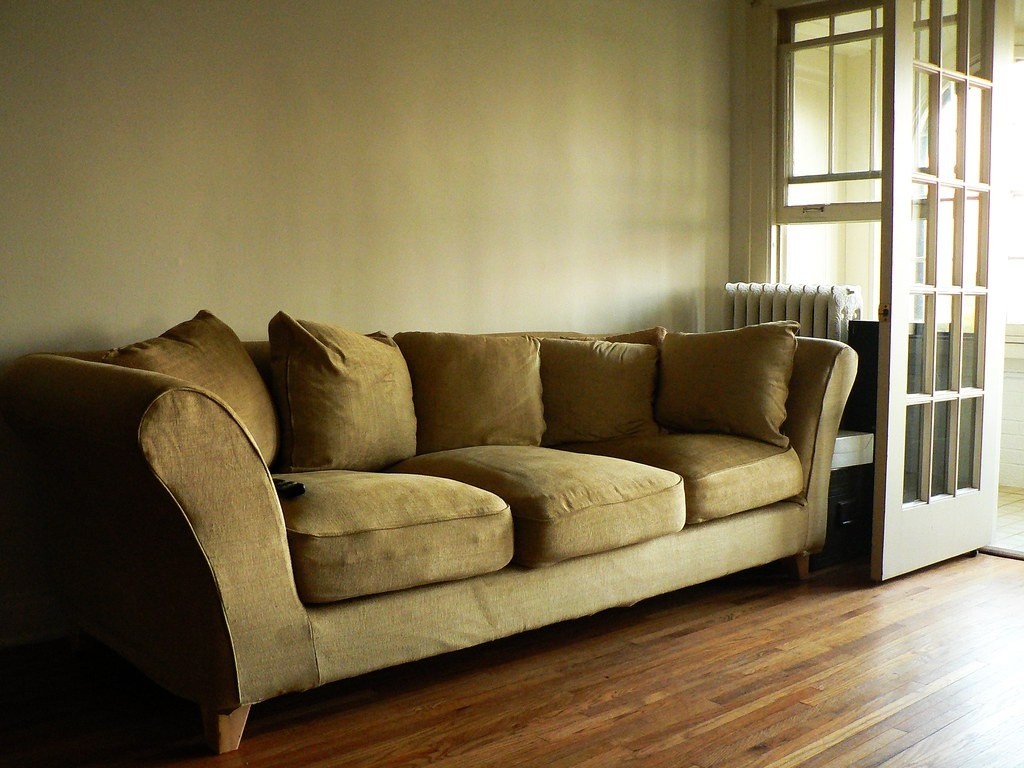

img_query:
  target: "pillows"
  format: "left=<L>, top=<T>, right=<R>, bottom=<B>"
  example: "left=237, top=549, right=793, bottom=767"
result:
left=392, top=330, right=548, bottom=452
left=102, top=308, right=281, bottom=471
left=536, top=336, right=658, bottom=443
left=655, top=318, right=800, bottom=449
left=267, top=310, right=418, bottom=473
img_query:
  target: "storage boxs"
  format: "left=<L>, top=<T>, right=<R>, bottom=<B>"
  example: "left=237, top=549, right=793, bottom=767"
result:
left=831, top=431, right=875, bottom=467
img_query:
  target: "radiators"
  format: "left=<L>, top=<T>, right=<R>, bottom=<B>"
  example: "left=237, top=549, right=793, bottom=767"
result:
left=722, top=281, right=862, bottom=340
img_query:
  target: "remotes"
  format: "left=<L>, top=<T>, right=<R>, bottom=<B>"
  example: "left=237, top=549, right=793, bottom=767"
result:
left=272, top=478, right=306, bottom=500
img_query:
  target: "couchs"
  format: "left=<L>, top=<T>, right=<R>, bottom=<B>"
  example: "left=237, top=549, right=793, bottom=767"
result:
left=0, top=335, right=860, bottom=755
